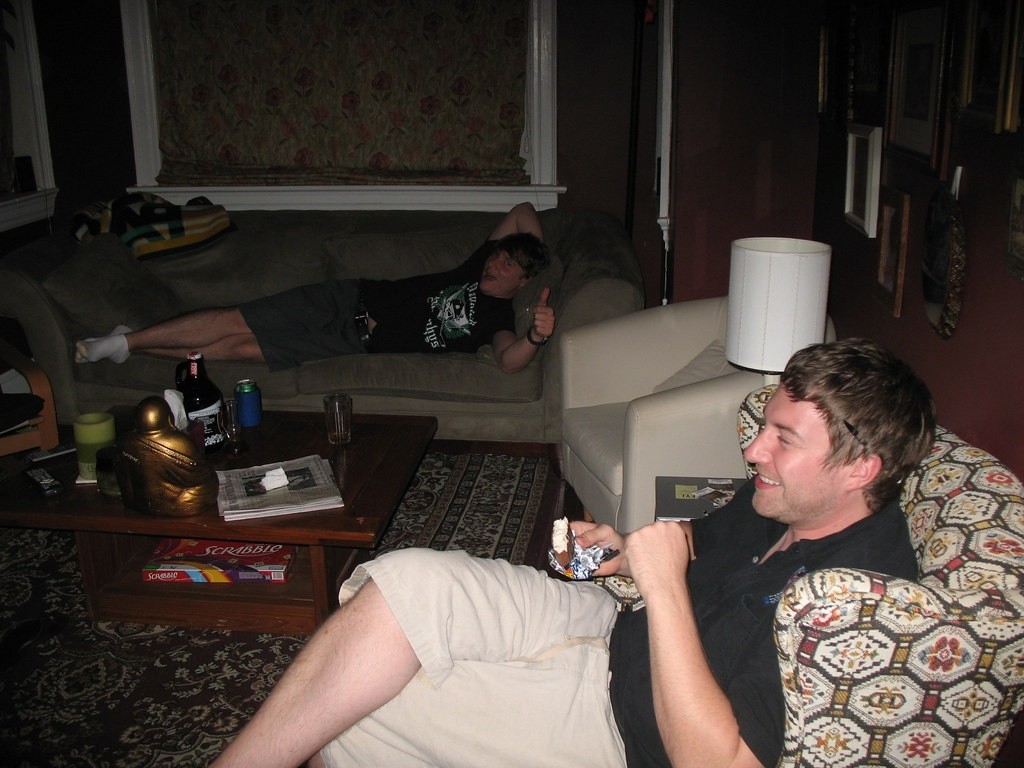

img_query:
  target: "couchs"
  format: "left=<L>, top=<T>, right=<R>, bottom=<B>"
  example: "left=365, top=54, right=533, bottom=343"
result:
left=597, top=383, right=1024, bottom=768
left=556, top=290, right=843, bottom=533
left=0, top=200, right=648, bottom=445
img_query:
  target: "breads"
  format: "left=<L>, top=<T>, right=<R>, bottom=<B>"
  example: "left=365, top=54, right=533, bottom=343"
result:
left=552, top=517, right=574, bottom=568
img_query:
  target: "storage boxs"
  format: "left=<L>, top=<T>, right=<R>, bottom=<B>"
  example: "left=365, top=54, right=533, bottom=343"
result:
left=142, top=538, right=299, bottom=588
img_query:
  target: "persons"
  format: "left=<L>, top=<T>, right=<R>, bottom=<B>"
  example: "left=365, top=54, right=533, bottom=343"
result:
left=75, top=202, right=556, bottom=374
left=119, top=395, right=219, bottom=515
left=208, top=336, right=937, bottom=767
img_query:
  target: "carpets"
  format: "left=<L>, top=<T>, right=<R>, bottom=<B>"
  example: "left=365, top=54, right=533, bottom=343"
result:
left=0, top=452, right=561, bottom=768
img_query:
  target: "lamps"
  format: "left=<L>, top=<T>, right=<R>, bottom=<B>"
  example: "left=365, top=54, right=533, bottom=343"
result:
left=724, top=229, right=833, bottom=386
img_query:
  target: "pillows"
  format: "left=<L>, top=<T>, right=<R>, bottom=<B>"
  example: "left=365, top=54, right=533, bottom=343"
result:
left=647, top=335, right=738, bottom=393
left=41, top=232, right=191, bottom=339
left=323, top=222, right=495, bottom=280
left=514, top=249, right=567, bottom=338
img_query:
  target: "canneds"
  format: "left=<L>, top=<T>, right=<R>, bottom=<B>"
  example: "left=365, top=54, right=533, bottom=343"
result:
left=234, top=379, right=259, bottom=392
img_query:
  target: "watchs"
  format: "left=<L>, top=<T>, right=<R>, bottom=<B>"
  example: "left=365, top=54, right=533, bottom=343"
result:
left=527, top=326, right=548, bottom=345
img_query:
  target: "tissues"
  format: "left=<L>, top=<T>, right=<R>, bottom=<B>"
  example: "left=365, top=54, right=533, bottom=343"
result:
left=164, top=389, right=205, bottom=455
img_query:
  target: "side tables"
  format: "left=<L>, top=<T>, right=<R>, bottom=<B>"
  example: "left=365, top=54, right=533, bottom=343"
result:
left=653, top=475, right=748, bottom=523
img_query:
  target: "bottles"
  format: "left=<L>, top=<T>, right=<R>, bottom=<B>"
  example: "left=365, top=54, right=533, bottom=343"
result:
left=174, top=351, right=228, bottom=454
left=217, top=400, right=241, bottom=457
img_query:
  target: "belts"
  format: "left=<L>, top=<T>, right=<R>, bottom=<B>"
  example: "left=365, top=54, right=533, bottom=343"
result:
left=353, top=276, right=374, bottom=355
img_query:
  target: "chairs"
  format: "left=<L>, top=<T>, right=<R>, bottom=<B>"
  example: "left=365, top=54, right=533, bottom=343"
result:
left=0, top=343, right=59, bottom=460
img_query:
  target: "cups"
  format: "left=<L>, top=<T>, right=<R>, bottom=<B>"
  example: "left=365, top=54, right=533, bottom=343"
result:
left=234, top=385, right=262, bottom=429
left=96, top=447, right=122, bottom=504
left=323, top=392, right=353, bottom=445
left=73, top=412, right=116, bottom=480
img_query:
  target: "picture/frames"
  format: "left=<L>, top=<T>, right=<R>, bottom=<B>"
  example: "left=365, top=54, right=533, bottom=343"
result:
left=803, top=0, right=1024, bottom=341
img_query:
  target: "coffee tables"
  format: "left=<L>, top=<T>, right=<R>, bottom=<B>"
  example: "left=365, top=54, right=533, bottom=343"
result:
left=0, top=405, right=442, bottom=629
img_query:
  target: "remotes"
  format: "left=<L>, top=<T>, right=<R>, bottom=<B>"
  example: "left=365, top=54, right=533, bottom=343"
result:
left=23, top=440, right=77, bottom=464
left=21, top=462, right=65, bottom=498
